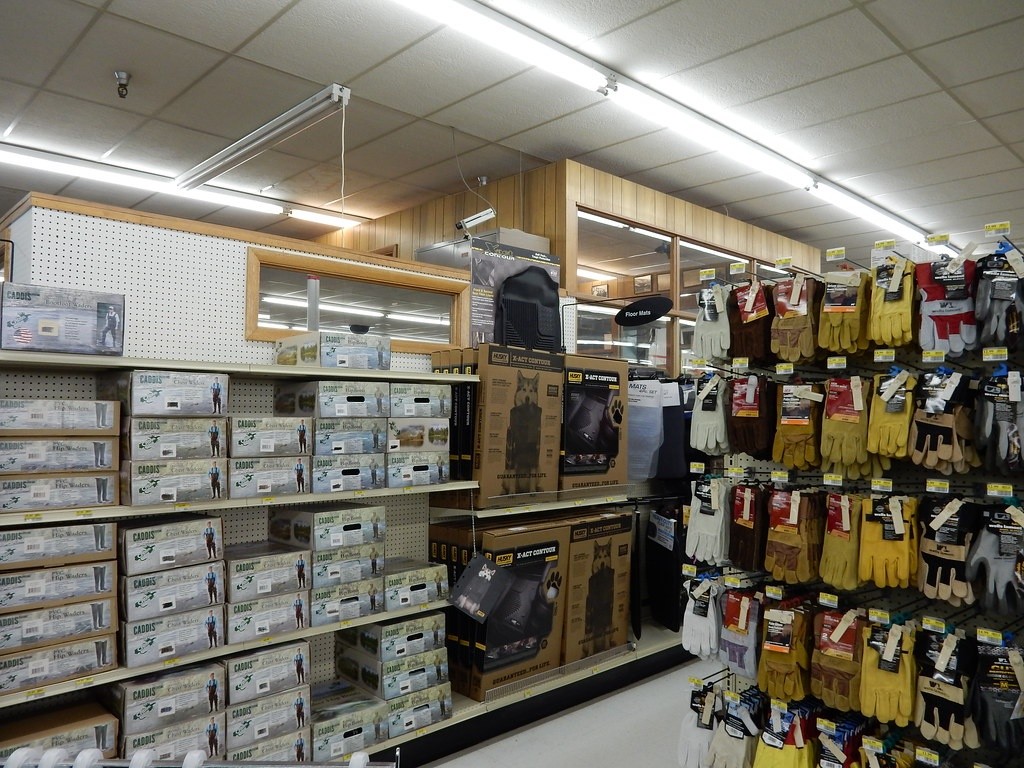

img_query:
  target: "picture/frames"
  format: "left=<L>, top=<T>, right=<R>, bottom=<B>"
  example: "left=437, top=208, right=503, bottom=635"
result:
left=591, top=284, right=609, bottom=298
left=632, top=273, right=653, bottom=294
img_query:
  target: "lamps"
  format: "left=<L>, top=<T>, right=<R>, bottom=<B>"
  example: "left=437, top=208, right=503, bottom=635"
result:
left=0, top=142, right=372, bottom=226
left=576, top=264, right=627, bottom=281
left=175, top=83, right=350, bottom=194
left=395, top=0, right=962, bottom=258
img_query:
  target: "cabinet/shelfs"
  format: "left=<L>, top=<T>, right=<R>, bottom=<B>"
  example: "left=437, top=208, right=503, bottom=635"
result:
left=0, top=348, right=686, bottom=763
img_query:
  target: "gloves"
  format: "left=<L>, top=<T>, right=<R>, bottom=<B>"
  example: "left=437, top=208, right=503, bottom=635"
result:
left=681, top=255, right=1024, bottom=768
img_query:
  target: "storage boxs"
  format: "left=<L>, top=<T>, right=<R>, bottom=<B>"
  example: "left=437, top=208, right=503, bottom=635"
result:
left=0, top=281, right=636, bottom=768
